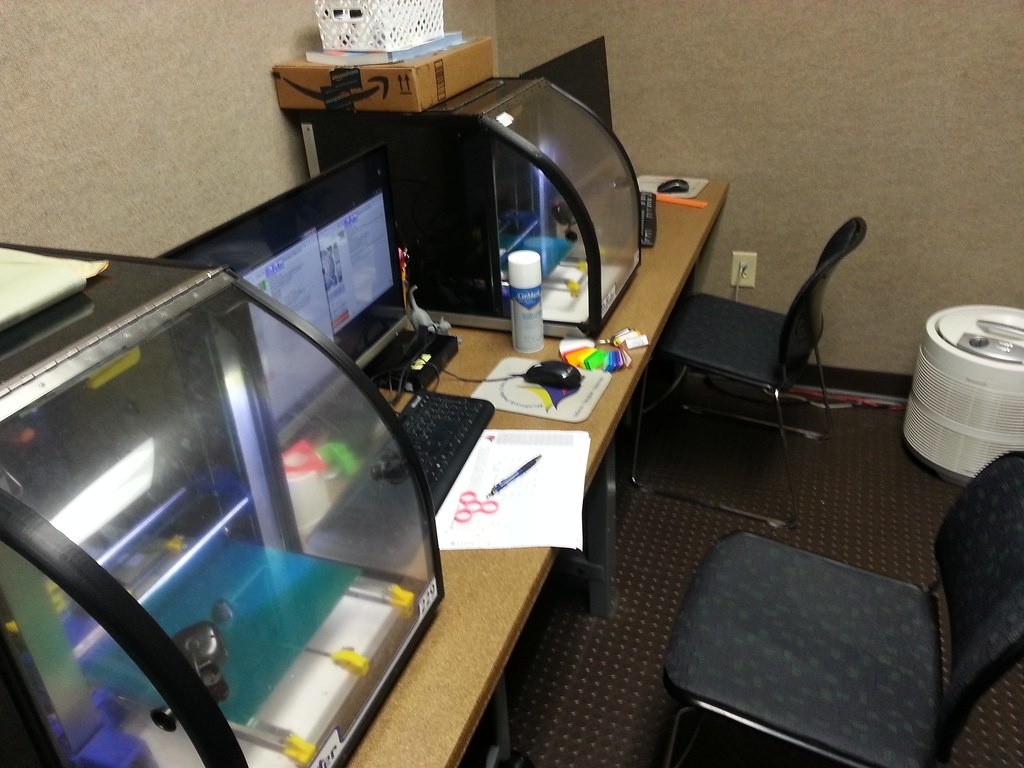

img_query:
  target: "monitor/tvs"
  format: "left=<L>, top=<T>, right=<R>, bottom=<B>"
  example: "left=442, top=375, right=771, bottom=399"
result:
left=150, top=140, right=408, bottom=443
left=519, top=35, right=612, bottom=133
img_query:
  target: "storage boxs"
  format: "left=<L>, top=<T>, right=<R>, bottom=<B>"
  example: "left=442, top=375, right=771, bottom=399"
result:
left=272, top=37, right=493, bottom=113
left=314, top=0, right=444, bottom=51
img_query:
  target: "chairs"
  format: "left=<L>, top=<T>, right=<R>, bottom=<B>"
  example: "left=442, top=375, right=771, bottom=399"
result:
left=630, top=215, right=867, bottom=523
left=662, top=450, right=1024, bottom=768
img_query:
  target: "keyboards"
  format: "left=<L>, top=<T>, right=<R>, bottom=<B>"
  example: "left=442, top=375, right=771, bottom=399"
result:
left=640, top=192, right=657, bottom=247
left=307, top=385, right=495, bottom=570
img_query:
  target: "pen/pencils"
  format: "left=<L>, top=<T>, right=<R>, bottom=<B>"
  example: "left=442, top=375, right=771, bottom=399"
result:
left=485, top=453, right=542, bottom=500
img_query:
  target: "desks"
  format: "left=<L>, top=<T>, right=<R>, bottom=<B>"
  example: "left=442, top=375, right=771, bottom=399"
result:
left=347, top=177, right=731, bottom=768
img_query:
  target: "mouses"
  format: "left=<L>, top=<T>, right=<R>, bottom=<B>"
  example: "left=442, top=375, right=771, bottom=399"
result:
left=525, top=359, right=582, bottom=388
left=658, top=179, right=690, bottom=193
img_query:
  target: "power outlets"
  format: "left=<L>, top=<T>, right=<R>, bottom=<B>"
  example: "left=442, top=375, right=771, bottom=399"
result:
left=731, top=251, right=758, bottom=288
